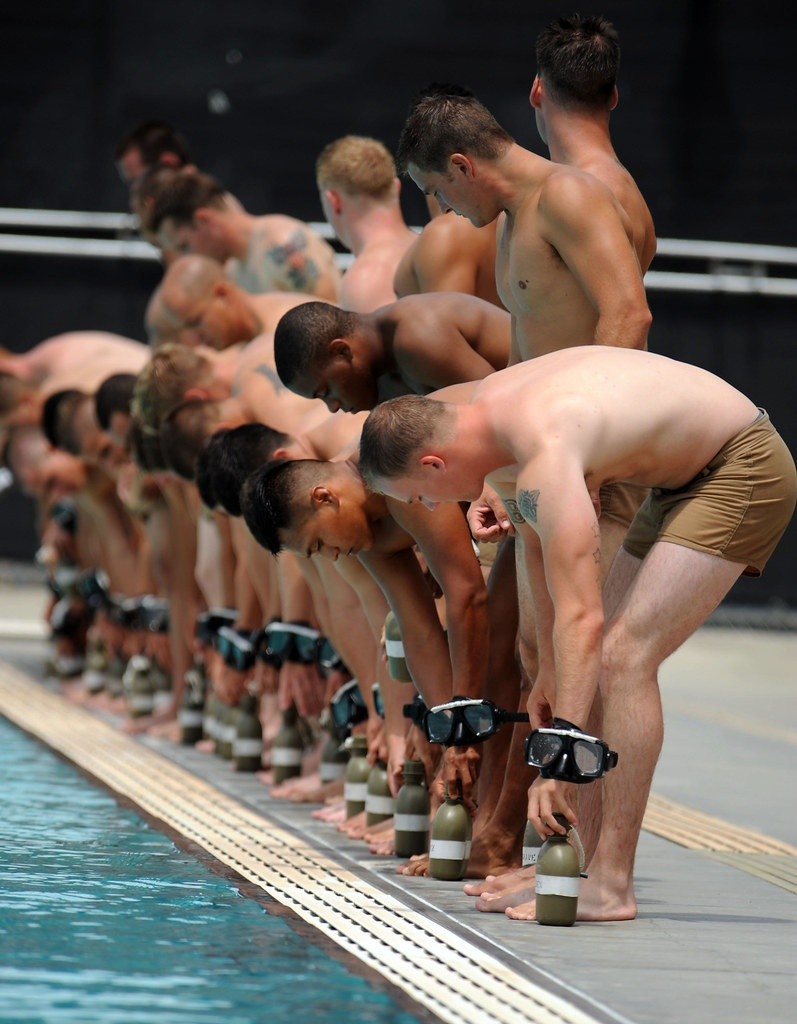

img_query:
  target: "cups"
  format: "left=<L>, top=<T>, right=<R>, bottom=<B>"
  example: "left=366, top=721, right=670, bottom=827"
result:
left=385, top=612, right=412, bottom=683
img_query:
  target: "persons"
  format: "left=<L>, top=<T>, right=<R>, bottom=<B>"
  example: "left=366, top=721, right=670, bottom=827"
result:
left=396, top=84, right=652, bottom=902
left=0, top=16, right=659, bottom=883
left=359, top=342, right=797, bottom=922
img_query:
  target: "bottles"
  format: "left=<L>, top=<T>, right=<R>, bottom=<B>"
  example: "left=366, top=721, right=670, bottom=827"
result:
left=321, top=720, right=347, bottom=785
left=344, top=735, right=368, bottom=820
left=233, top=695, right=261, bottom=771
left=428, top=782, right=468, bottom=878
left=271, top=708, right=301, bottom=780
left=43, top=637, right=235, bottom=759
left=395, top=762, right=427, bottom=858
left=367, top=762, right=394, bottom=825
left=536, top=813, right=578, bottom=925
